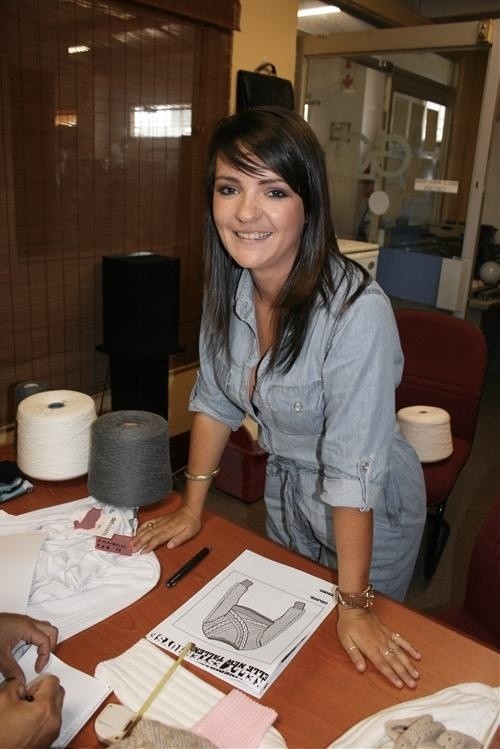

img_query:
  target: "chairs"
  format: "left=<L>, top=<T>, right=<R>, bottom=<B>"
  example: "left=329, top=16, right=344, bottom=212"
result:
left=434, top=493, right=500, bottom=653
left=390, top=307, right=485, bottom=583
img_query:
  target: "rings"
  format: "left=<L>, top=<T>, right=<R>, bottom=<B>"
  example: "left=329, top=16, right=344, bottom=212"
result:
left=346, top=645, right=356, bottom=654
left=147, top=523, right=153, bottom=529
left=391, top=633, right=400, bottom=640
left=382, top=649, right=392, bottom=657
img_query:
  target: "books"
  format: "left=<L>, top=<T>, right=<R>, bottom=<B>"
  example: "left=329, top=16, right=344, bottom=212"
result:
left=0, top=640, right=112, bottom=749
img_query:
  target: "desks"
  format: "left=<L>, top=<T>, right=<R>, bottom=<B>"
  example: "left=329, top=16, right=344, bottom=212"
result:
left=0, top=442, right=500, bottom=748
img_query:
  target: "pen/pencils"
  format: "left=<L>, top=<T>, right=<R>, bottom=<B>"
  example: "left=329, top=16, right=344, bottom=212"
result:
left=20, top=695, right=34, bottom=701
left=166, top=547, right=210, bottom=588
left=121, top=642, right=193, bottom=740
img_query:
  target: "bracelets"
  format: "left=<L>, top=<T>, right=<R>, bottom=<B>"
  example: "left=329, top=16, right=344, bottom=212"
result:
left=335, top=585, right=376, bottom=609
left=182, top=468, right=220, bottom=481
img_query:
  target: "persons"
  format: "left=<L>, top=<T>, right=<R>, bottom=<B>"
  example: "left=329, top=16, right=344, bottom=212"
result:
left=0, top=612, right=63, bottom=749
left=131, top=104, right=427, bottom=690
